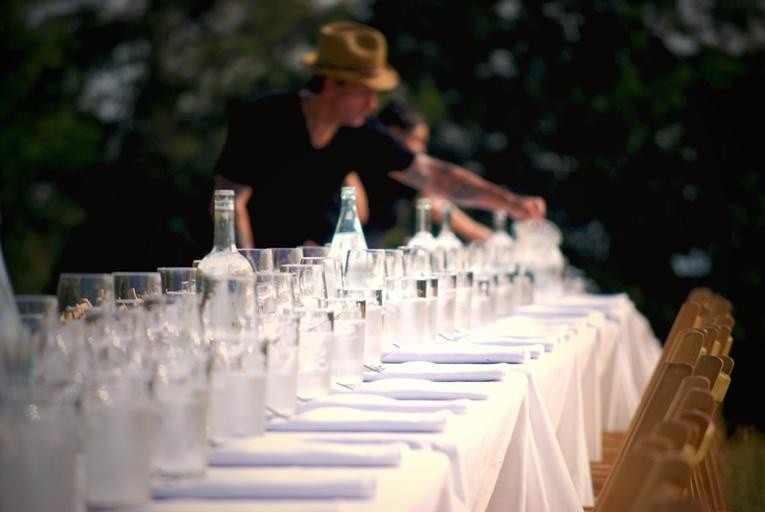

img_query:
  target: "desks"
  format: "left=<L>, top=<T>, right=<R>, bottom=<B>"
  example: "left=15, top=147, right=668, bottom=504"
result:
left=139, top=292, right=663, bottom=511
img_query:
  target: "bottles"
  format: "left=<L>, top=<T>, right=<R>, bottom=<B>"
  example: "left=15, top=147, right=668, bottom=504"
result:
left=328, top=186, right=370, bottom=251
left=484, top=209, right=516, bottom=243
left=195, top=190, right=254, bottom=298
left=408, top=197, right=437, bottom=247
left=436, top=203, right=463, bottom=247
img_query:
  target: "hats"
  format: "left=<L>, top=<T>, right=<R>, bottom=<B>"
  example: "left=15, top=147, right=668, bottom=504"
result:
left=298, top=19, right=402, bottom=93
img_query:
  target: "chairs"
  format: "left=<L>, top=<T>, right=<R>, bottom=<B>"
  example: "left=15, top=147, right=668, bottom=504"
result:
left=582, top=288, right=737, bottom=510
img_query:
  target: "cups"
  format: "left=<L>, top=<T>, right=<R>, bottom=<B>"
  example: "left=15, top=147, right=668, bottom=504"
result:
left=0, top=243, right=585, bottom=511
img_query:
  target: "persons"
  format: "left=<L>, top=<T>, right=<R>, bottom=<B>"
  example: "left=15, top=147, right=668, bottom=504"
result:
left=285, top=97, right=493, bottom=250
left=188, top=19, right=548, bottom=250
